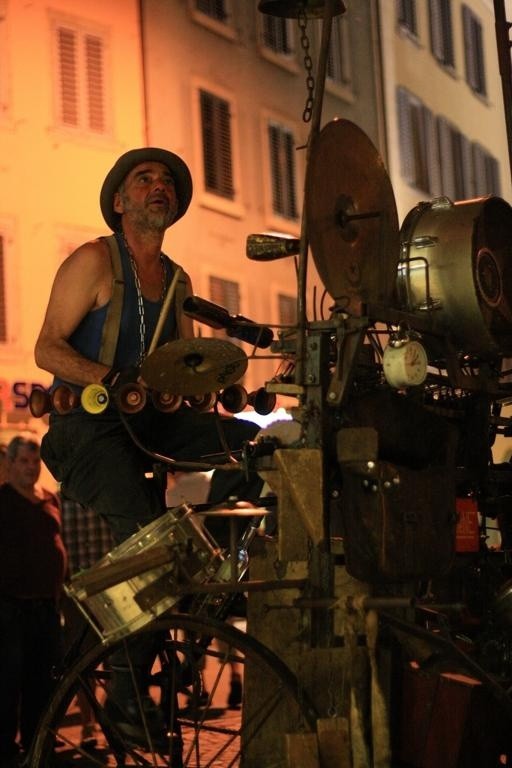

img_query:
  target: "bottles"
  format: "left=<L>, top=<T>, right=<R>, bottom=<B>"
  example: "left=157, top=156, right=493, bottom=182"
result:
left=196, top=526, right=257, bottom=620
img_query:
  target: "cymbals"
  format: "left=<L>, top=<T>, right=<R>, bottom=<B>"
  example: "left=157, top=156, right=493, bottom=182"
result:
left=305, top=117, right=399, bottom=315
left=140, top=336, right=249, bottom=397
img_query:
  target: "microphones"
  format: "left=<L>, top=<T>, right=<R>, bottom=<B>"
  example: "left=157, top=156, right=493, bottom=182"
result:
left=182, top=294, right=274, bottom=350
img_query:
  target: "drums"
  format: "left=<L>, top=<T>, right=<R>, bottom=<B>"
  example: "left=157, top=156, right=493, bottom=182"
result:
left=398, top=199, right=512, bottom=358
left=64, top=503, right=227, bottom=645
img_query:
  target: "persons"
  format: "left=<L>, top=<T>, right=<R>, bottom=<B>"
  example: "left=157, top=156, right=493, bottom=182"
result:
left=0, top=435, right=67, bottom=768
left=184, top=642, right=243, bottom=711
left=34, top=147, right=196, bottom=754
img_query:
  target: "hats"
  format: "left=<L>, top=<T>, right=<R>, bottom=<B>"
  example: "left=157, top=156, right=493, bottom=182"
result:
left=100, top=148, right=193, bottom=232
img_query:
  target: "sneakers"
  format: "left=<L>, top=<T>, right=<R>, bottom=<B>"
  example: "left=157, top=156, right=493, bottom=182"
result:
left=104, top=697, right=174, bottom=754
left=187, top=687, right=208, bottom=706
left=229, top=681, right=243, bottom=705
